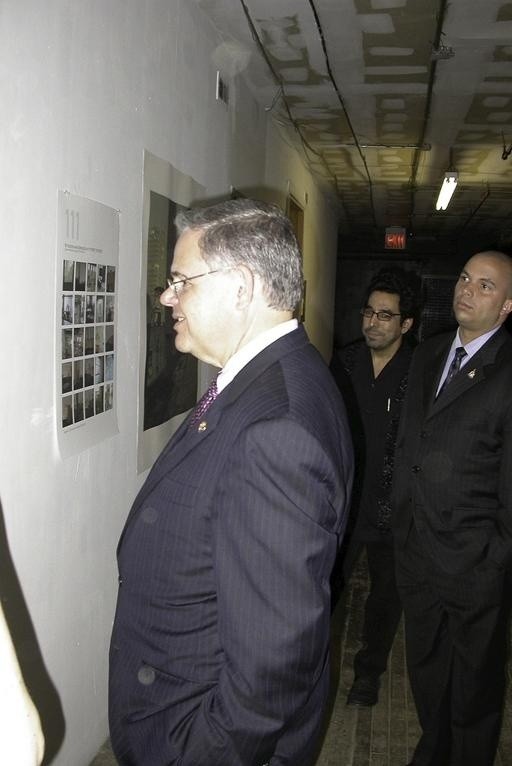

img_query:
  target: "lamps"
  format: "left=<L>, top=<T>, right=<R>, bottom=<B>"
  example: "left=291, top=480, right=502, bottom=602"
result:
left=435, top=146, right=459, bottom=210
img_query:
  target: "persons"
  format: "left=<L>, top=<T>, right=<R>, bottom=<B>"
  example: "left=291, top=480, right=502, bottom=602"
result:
left=384, top=246, right=511, bottom=766
left=332, top=271, right=430, bottom=713
left=101, top=193, right=356, bottom=766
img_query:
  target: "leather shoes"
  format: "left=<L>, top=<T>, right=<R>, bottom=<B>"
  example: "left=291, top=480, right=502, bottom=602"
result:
left=348, top=675, right=379, bottom=707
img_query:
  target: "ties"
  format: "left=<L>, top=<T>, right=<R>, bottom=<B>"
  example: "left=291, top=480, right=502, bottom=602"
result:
left=188, top=372, right=222, bottom=433
left=434, top=346, right=467, bottom=405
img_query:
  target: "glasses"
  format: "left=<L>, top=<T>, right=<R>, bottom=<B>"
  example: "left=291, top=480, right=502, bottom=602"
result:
left=360, top=309, right=402, bottom=321
left=167, top=269, right=240, bottom=298
